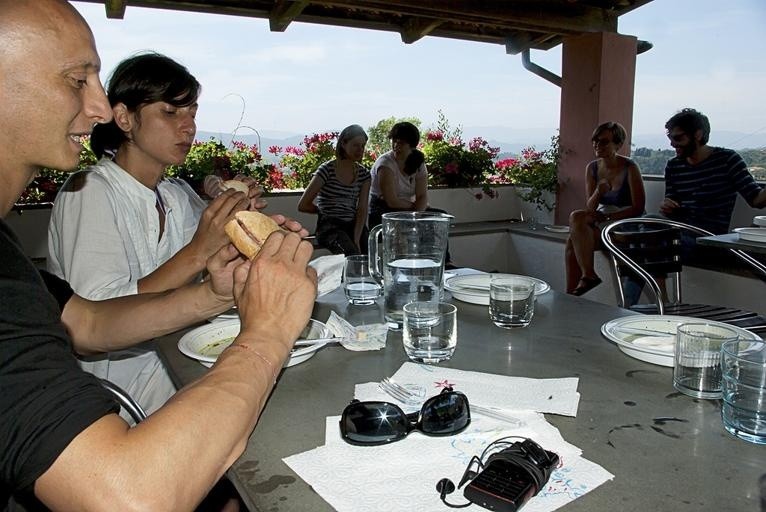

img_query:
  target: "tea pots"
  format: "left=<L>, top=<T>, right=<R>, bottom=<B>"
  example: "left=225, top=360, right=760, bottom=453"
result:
left=365, top=209, right=457, bottom=328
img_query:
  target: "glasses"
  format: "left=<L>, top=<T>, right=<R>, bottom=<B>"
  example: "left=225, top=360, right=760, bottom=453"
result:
left=593, top=137, right=614, bottom=146
left=338, top=387, right=471, bottom=447
left=667, top=132, right=686, bottom=142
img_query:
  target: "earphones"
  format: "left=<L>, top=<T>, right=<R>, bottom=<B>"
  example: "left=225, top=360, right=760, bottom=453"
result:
left=436, top=475, right=455, bottom=498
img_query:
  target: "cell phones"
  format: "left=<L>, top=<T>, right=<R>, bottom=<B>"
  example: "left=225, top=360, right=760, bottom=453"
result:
left=464, top=438, right=560, bottom=512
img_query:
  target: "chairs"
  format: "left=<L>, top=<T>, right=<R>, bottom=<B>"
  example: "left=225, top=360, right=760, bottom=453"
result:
left=600, top=216, right=766, bottom=341
left=91, top=375, right=146, bottom=425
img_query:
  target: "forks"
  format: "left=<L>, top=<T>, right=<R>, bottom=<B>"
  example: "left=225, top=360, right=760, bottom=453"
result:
left=374, top=379, right=532, bottom=429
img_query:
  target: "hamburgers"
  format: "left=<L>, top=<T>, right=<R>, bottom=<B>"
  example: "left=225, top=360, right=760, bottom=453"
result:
left=224, top=210, right=295, bottom=264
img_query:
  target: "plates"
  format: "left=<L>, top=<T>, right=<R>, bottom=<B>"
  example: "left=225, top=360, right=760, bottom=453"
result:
left=753, top=215, right=766, bottom=227
left=601, top=312, right=764, bottom=370
left=443, top=272, right=551, bottom=305
left=734, top=228, right=766, bottom=242
left=179, top=319, right=331, bottom=373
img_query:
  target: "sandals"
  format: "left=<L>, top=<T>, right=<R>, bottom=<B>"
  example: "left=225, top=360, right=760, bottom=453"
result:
left=573, top=273, right=602, bottom=296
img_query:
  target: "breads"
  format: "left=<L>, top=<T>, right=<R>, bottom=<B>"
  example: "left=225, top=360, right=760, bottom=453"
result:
left=219, top=179, right=254, bottom=197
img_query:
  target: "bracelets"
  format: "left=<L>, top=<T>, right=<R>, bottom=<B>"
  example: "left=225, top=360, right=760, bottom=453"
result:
left=225, top=344, right=276, bottom=385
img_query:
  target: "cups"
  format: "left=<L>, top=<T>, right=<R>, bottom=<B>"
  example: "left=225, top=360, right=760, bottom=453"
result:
left=672, top=320, right=741, bottom=399
left=342, top=254, right=386, bottom=307
left=528, top=216, right=537, bottom=231
left=488, top=274, right=539, bottom=328
left=719, top=337, right=766, bottom=442
left=402, top=300, right=458, bottom=364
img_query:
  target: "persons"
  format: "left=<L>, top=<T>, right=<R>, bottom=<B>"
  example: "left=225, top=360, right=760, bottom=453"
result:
left=565, top=122, right=646, bottom=296
left=618, top=109, right=766, bottom=307
left=366, top=121, right=452, bottom=267
left=0, top=1, right=319, bottom=511
left=297, top=124, right=372, bottom=256
left=46, top=54, right=268, bottom=427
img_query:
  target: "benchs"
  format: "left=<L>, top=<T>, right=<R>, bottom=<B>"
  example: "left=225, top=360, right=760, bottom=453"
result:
left=511, top=215, right=765, bottom=326
left=30, top=217, right=509, bottom=312
